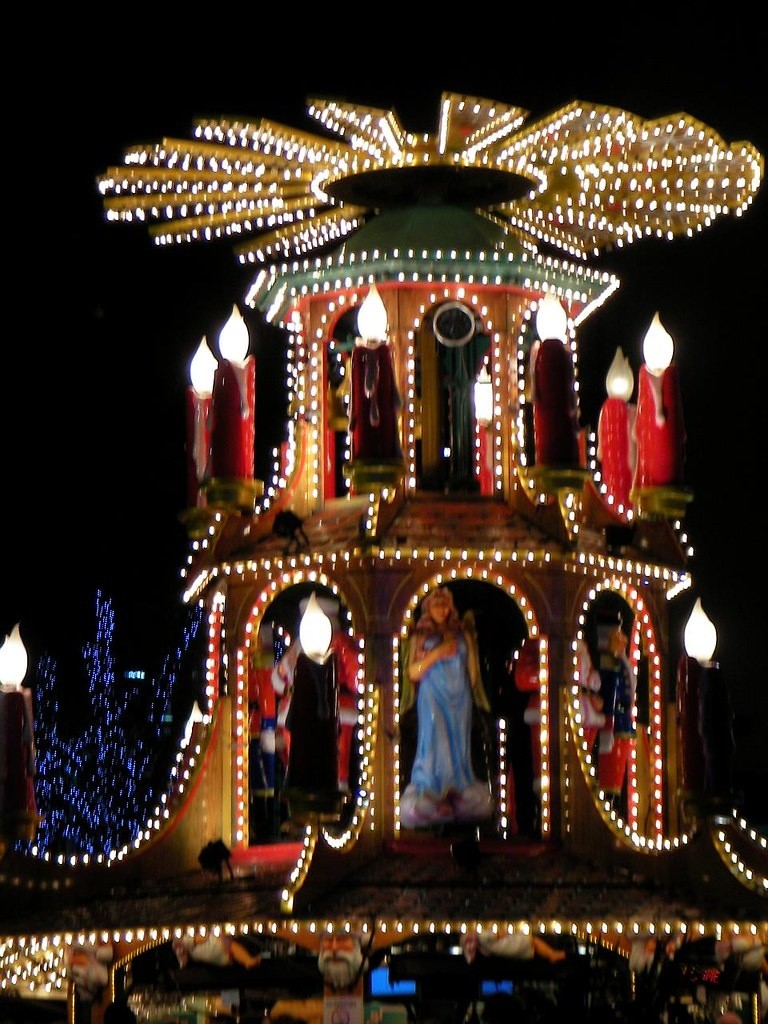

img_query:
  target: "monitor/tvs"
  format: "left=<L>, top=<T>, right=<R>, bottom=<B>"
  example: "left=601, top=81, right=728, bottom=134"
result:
left=369, top=967, right=416, bottom=997
left=482, top=980, right=513, bottom=996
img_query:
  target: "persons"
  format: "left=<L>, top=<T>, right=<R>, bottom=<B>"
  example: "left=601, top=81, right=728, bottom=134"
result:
left=69, top=941, right=113, bottom=1001
left=173, top=936, right=261, bottom=967
left=406, top=588, right=480, bottom=795
left=517, top=619, right=638, bottom=815
left=318, top=932, right=362, bottom=988
left=248, top=598, right=360, bottom=828
left=460, top=931, right=565, bottom=962
